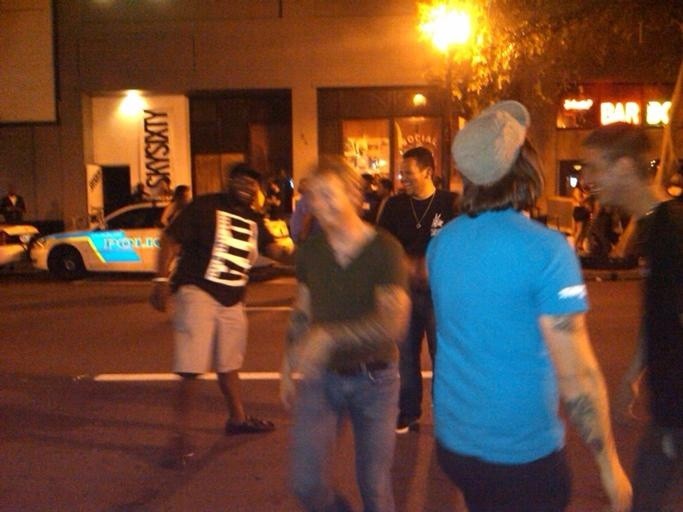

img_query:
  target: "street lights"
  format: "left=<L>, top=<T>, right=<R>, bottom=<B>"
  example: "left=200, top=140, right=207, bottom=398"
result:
left=422, top=5, right=481, bottom=194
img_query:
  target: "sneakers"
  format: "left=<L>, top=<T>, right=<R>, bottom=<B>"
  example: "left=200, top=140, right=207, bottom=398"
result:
left=224, top=416, right=275, bottom=435
left=395, top=413, right=422, bottom=433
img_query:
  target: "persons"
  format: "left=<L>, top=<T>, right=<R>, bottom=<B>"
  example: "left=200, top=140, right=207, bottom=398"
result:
left=132, top=176, right=189, bottom=227
left=272, top=170, right=314, bottom=241
left=424, top=99, right=634, bottom=511
left=575, top=195, right=647, bottom=281
left=578, top=121, right=683, bottom=512
left=377, top=146, right=460, bottom=437
left=1, top=183, right=27, bottom=220
left=649, top=64, right=683, bottom=202
left=277, top=154, right=412, bottom=511
left=359, top=173, right=393, bottom=224
left=149, top=160, right=295, bottom=461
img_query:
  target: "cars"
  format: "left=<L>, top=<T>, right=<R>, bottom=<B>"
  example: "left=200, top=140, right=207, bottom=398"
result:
left=0, top=224, right=37, bottom=267
left=30, top=191, right=295, bottom=282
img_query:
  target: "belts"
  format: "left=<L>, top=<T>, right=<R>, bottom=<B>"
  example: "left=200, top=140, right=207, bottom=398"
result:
left=328, top=360, right=396, bottom=378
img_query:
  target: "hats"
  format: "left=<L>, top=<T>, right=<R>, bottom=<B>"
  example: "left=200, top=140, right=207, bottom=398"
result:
left=451, top=99, right=532, bottom=188
left=230, top=163, right=264, bottom=186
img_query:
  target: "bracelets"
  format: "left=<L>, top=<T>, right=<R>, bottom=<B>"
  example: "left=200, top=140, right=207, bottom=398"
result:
left=151, top=276, right=170, bottom=283
